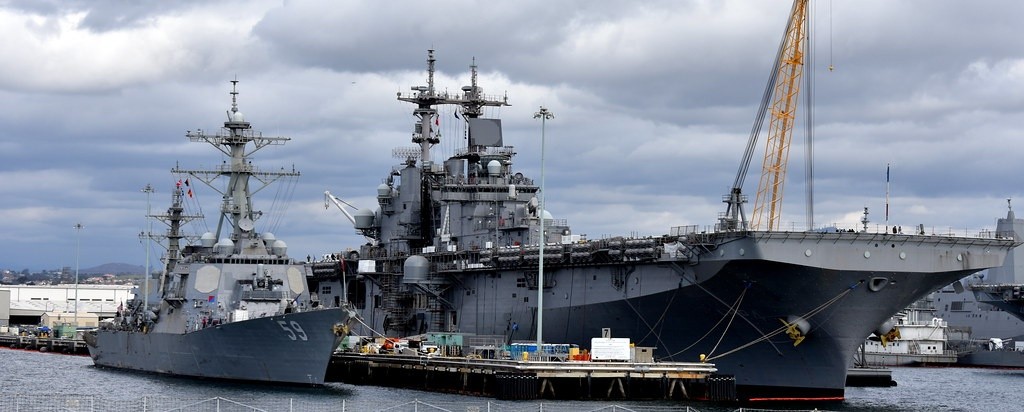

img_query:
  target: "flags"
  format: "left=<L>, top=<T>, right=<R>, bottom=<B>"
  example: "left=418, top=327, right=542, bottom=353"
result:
left=185, top=178, right=189, bottom=186
left=176, top=179, right=182, bottom=186
left=188, top=189, right=192, bottom=198
left=436, top=116, right=438, bottom=125
left=454, top=111, right=459, bottom=119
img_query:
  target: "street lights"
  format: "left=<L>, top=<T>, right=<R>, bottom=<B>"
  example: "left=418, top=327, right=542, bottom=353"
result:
left=72, top=222, right=85, bottom=339
left=141, top=184, right=155, bottom=308
left=533, top=105, right=554, bottom=353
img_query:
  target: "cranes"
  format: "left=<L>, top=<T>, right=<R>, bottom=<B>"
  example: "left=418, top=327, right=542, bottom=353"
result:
left=715, top=0, right=834, bottom=232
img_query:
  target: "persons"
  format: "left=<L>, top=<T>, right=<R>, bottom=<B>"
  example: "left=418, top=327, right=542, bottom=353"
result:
left=522, top=350, right=529, bottom=361
left=202, top=316, right=206, bottom=328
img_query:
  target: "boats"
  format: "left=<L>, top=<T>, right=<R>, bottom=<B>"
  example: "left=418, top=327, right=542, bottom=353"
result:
left=845, top=163, right=1023, bottom=386
left=308, top=46, right=1023, bottom=409
left=84, top=71, right=359, bottom=385
